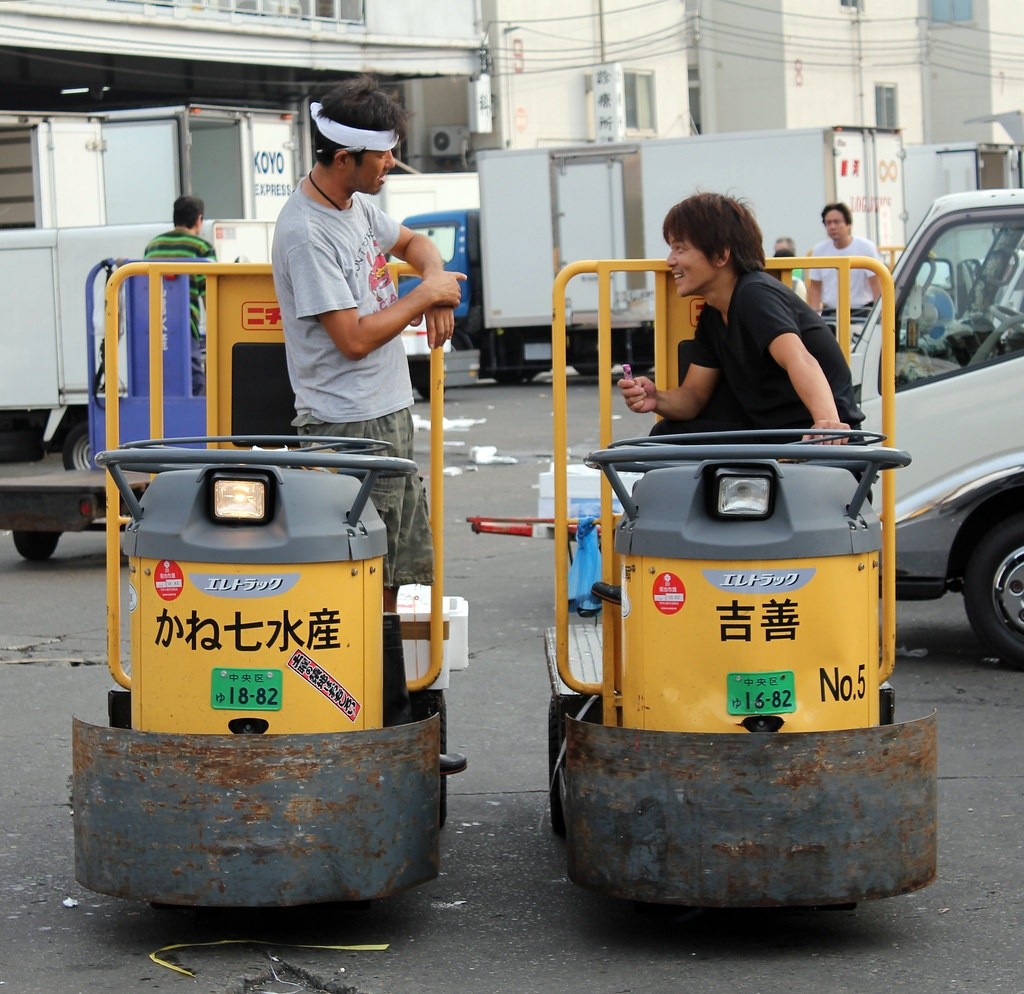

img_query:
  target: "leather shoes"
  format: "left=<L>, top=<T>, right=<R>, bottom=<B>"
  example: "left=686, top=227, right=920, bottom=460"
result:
left=591, top=582, right=622, bottom=605
left=439, top=753, right=468, bottom=775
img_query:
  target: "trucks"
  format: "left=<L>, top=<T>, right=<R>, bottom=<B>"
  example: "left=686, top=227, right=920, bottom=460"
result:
left=1, top=96, right=486, bottom=561
left=863, top=184, right=1024, bottom=666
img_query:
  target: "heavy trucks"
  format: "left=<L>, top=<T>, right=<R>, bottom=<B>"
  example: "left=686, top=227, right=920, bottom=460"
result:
left=399, top=126, right=1024, bottom=377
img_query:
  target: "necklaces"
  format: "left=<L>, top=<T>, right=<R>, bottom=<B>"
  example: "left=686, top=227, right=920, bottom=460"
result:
left=308, top=170, right=344, bottom=212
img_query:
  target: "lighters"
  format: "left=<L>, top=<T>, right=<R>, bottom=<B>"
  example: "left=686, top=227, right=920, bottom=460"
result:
left=622, top=364, right=633, bottom=381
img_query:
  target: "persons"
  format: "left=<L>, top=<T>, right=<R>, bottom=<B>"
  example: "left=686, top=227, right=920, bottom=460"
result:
left=144, top=195, right=223, bottom=395
left=774, top=203, right=885, bottom=319
left=591, top=192, right=873, bottom=605
left=272, top=75, right=471, bottom=777
